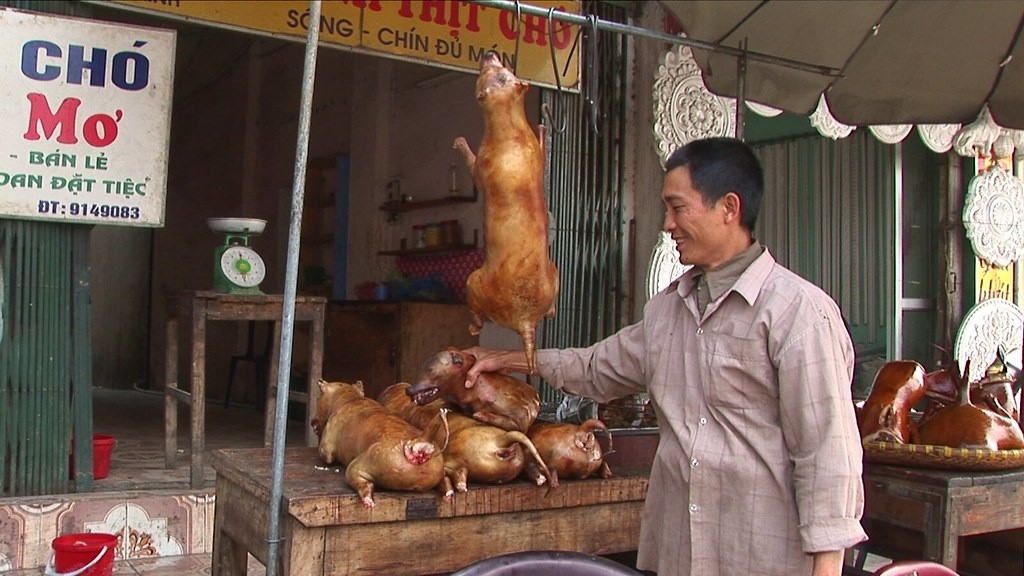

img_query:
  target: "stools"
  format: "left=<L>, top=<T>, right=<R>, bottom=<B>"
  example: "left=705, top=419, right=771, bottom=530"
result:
left=164, top=289, right=327, bottom=492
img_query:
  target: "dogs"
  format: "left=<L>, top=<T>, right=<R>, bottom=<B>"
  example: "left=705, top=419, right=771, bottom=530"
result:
left=310, top=347, right=614, bottom=508
left=454, top=51, right=560, bottom=375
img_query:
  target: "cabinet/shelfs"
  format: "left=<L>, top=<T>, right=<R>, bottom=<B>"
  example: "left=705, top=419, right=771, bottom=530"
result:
left=324, top=298, right=478, bottom=403
left=375, top=195, right=479, bottom=256
left=296, top=153, right=350, bottom=300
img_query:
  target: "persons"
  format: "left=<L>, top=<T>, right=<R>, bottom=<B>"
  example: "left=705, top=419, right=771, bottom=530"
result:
left=456, top=136, right=868, bottom=575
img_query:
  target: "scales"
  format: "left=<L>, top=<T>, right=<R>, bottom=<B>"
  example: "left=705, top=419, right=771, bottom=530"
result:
left=206, top=217, right=269, bottom=295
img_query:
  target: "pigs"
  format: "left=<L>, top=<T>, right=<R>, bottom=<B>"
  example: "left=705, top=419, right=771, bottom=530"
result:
left=856, top=345, right=1024, bottom=451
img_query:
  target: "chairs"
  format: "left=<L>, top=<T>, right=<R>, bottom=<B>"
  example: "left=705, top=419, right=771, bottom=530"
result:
left=226, top=320, right=276, bottom=412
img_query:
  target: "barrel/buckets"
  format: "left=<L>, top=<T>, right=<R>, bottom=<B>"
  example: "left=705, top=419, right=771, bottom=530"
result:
left=44, top=532, right=118, bottom=576
left=67, top=434, right=116, bottom=479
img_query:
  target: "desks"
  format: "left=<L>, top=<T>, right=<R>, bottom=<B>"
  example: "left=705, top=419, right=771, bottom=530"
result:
left=849, top=459, right=1024, bottom=576
left=207, top=446, right=653, bottom=576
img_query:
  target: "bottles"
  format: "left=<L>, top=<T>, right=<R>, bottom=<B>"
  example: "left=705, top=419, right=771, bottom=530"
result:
left=412, top=219, right=465, bottom=249
left=353, top=280, right=390, bottom=303
